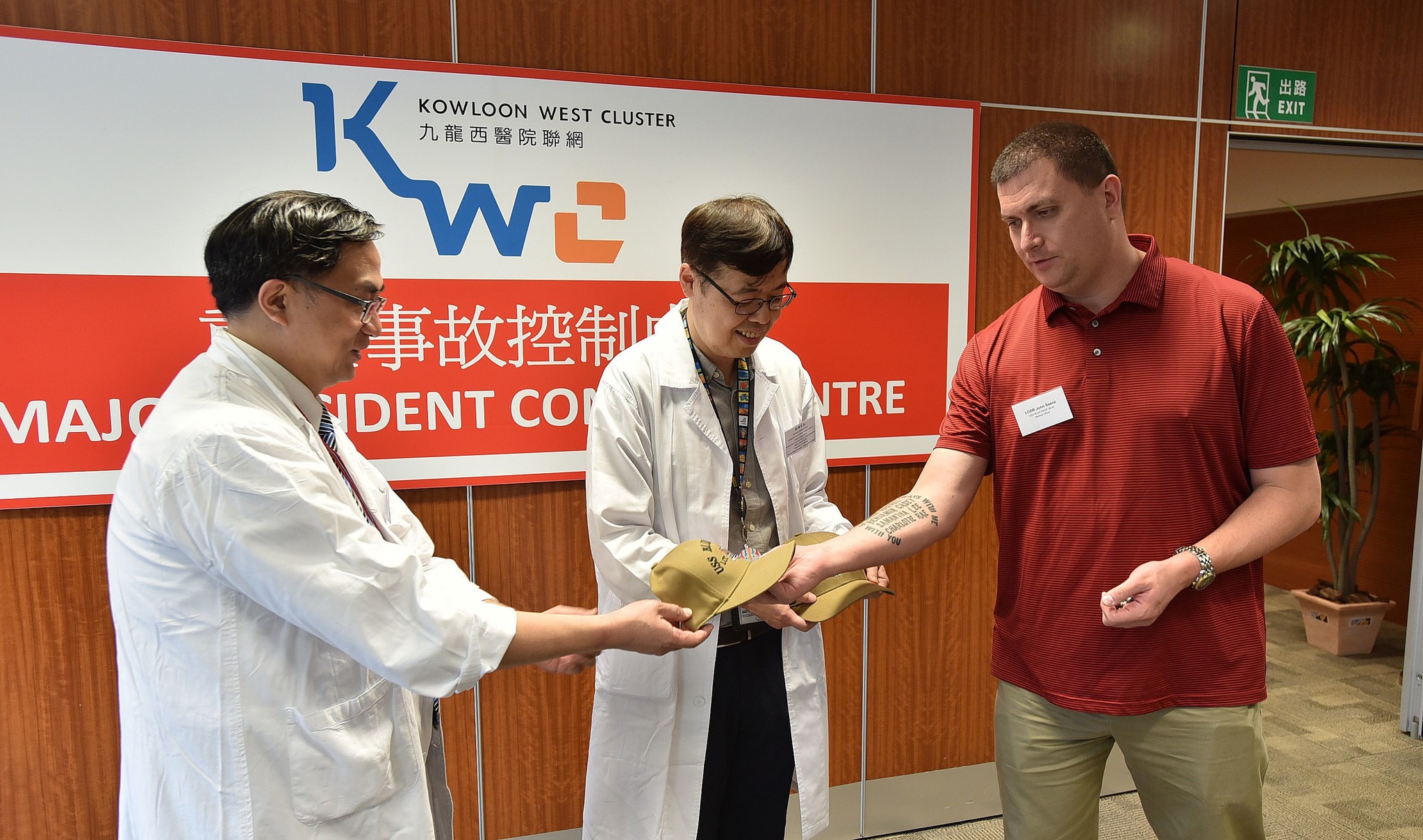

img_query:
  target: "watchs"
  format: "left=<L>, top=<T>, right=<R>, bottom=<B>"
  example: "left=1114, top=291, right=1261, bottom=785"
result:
left=1172, top=545, right=1215, bottom=591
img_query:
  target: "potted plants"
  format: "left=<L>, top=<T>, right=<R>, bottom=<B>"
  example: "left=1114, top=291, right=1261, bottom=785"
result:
left=1229, top=194, right=1422, bottom=659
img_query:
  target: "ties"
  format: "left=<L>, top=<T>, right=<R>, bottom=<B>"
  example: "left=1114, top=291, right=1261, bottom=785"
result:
left=317, top=404, right=377, bottom=529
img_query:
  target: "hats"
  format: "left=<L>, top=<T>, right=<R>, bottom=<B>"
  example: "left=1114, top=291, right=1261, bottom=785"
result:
left=649, top=540, right=796, bottom=634
left=782, top=529, right=898, bottom=622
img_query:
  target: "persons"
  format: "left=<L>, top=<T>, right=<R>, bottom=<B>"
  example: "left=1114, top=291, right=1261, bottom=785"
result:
left=581, top=194, right=890, bottom=840
left=105, top=189, right=715, bottom=840
left=768, top=119, right=1323, bottom=840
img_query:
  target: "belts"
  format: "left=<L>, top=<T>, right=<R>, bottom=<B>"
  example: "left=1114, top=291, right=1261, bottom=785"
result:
left=713, top=622, right=776, bottom=649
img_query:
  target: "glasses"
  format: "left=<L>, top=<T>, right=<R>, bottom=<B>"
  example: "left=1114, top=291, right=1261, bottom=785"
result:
left=686, top=265, right=796, bottom=316
left=280, top=274, right=389, bottom=324
